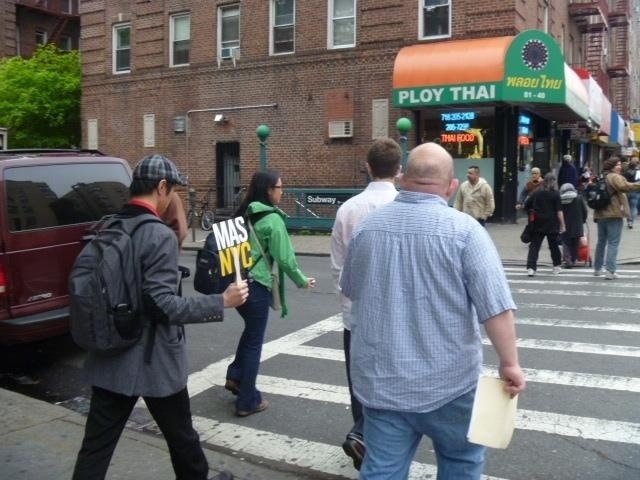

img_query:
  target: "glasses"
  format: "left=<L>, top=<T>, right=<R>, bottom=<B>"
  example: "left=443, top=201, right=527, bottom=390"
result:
left=531, top=172, right=539, bottom=175
left=271, top=184, right=285, bottom=190
left=616, top=162, right=623, bottom=168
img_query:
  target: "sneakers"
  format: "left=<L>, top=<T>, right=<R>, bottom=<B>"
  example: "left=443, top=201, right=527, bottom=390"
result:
left=225, top=376, right=241, bottom=395
left=604, top=269, right=618, bottom=280
left=527, top=267, right=536, bottom=276
left=553, top=265, right=563, bottom=275
left=593, top=268, right=604, bottom=277
left=238, top=398, right=270, bottom=416
left=626, top=219, right=635, bottom=229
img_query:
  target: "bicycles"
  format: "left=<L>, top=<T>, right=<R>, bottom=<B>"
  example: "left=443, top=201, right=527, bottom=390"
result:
left=184, top=198, right=215, bottom=230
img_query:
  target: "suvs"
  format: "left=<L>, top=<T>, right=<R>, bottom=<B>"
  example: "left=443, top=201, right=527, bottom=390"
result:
left=0, top=148, right=135, bottom=345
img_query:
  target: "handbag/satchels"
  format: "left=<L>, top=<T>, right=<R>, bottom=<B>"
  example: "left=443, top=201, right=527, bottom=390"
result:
left=520, top=222, right=535, bottom=244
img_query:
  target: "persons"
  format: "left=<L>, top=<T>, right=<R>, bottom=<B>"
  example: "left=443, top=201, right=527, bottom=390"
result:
left=514, top=167, right=547, bottom=209
left=557, top=154, right=578, bottom=189
left=338, top=142, right=526, bottom=480
left=222, top=169, right=315, bottom=417
left=454, top=165, right=494, bottom=226
left=619, top=154, right=630, bottom=176
left=327, top=139, right=403, bottom=471
left=525, top=172, right=567, bottom=279
left=591, top=156, right=640, bottom=280
left=71, top=154, right=250, bottom=480
left=558, top=183, right=587, bottom=269
left=622, top=157, right=640, bottom=229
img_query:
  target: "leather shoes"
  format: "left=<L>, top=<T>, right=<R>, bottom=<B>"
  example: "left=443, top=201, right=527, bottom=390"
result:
left=208, top=470, right=233, bottom=480
left=342, top=438, right=366, bottom=472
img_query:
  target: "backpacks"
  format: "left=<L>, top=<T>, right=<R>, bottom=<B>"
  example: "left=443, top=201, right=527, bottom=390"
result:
left=68, top=214, right=179, bottom=354
left=584, top=171, right=621, bottom=209
left=196, top=206, right=278, bottom=296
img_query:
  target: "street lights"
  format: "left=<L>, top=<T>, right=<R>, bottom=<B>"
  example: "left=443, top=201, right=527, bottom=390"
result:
left=255, top=126, right=269, bottom=167
left=396, top=118, right=411, bottom=173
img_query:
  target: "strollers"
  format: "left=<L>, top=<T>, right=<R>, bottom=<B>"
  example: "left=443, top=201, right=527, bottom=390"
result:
left=576, top=219, right=592, bottom=267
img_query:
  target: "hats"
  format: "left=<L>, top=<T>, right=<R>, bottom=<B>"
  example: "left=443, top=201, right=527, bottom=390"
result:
left=563, top=155, right=573, bottom=162
left=131, top=152, right=190, bottom=186
